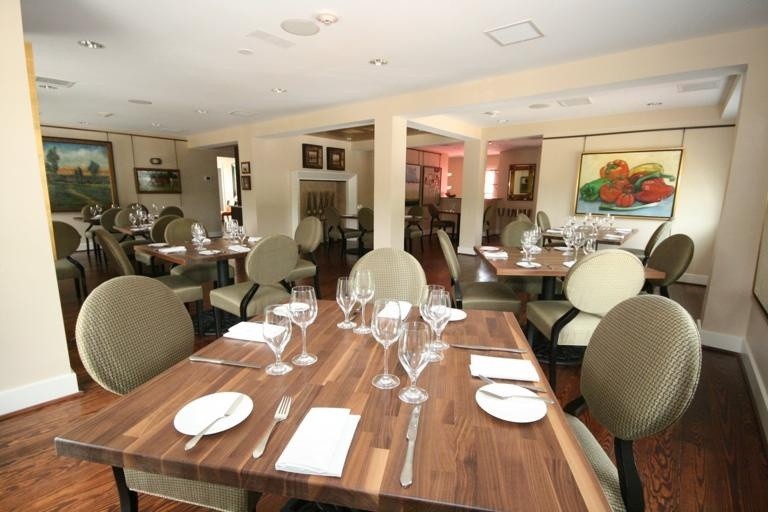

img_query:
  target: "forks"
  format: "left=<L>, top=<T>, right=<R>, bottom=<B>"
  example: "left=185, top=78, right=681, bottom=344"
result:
left=478, top=374, right=546, bottom=396
left=184, top=392, right=245, bottom=450
left=253, top=396, right=293, bottom=460
left=480, top=390, right=554, bottom=408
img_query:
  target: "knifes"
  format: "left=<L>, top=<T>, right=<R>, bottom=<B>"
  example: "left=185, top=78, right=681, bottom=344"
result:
left=452, top=344, right=527, bottom=353
left=190, top=355, right=261, bottom=372
left=400, top=405, right=423, bottom=486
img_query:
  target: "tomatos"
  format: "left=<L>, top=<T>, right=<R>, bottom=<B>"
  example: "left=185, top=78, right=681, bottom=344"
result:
left=599, top=183, right=621, bottom=203
left=616, top=189, right=634, bottom=207
left=612, top=174, right=630, bottom=188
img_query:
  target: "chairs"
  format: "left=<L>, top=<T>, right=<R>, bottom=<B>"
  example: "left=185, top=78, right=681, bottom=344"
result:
left=75, top=273, right=265, bottom=512
left=498, top=219, right=545, bottom=298
left=646, top=230, right=694, bottom=300
left=536, top=210, right=552, bottom=244
left=619, top=219, right=672, bottom=266
left=563, top=296, right=706, bottom=511
left=323, top=205, right=499, bottom=263
left=50, top=205, right=321, bottom=339
left=436, top=227, right=522, bottom=318
left=526, top=249, right=646, bottom=393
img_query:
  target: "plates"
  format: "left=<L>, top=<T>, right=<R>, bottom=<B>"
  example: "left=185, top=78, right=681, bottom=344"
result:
left=475, top=384, right=548, bottom=424
left=449, top=307, right=467, bottom=322
left=200, top=249, right=221, bottom=256
left=174, top=393, right=252, bottom=436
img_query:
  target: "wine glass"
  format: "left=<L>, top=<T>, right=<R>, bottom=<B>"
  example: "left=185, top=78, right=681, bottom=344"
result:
left=129, top=202, right=164, bottom=228
left=191, top=223, right=207, bottom=252
left=224, top=219, right=246, bottom=246
left=562, top=211, right=615, bottom=265
left=89, top=203, right=102, bottom=216
left=518, top=228, right=541, bottom=266
left=263, top=271, right=451, bottom=405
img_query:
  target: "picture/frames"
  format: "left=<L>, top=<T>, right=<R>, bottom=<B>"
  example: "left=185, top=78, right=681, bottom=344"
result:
left=240, top=175, right=251, bottom=190
left=134, top=167, right=182, bottom=194
left=240, top=161, right=251, bottom=174
left=507, top=163, right=537, bottom=202
left=571, top=146, right=684, bottom=223
left=42, top=137, right=120, bottom=212
left=422, top=165, right=442, bottom=205
left=326, top=146, right=345, bottom=172
left=301, top=144, right=324, bottom=169
left=406, top=164, right=421, bottom=183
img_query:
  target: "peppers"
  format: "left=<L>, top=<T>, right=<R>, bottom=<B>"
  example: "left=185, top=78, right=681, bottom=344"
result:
left=580, top=178, right=611, bottom=202
left=628, top=174, right=674, bottom=203
left=628, top=163, right=675, bottom=182
left=600, top=160, right=629, bottom=182
